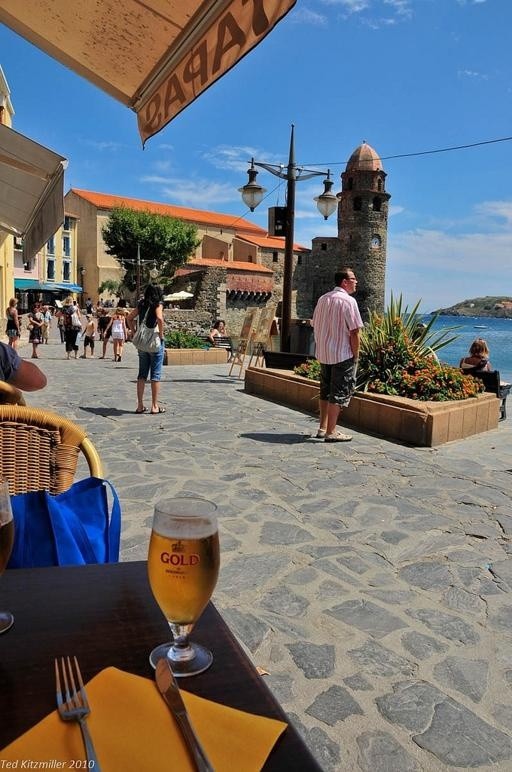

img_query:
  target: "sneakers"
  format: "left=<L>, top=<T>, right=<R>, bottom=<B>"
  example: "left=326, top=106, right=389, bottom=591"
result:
left=317, top=429, right=327, bottom=437
left=79, top=356, right=86, bottom=358
left=112, top=356, right=121, bottom=362
left=325, top=431, right=353, bottom=442
left=99, top=357, right=104, bottom=359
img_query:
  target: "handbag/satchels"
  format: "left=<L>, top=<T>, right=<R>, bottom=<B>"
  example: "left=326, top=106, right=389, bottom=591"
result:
left=71, top=312, right=81, bottom=327
left=6, top=475, right=122, bottom=570
left=133, top=320, right=161, bottom=353
left=26, top=323, right=33, bottom=331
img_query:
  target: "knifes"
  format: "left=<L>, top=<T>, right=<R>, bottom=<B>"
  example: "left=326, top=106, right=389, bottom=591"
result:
left=153, top=655, right=214, bottom=771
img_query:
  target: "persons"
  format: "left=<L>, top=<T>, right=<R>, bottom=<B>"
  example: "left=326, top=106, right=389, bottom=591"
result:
left=459, top=339, right=493, bottom=373
left=27, top=295, right=126, bottom=362
left=313, top=267, right=364, bottom=442
left=209, top=319, right=229, bottom=348
left=6, top=298, right=20, bottom=351
left=0, top=342, right=48, bottom=409
left=127, top=285, right=167, bottom=414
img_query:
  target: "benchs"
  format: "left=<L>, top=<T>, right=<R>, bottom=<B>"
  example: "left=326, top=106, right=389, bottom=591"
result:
left=195, top=335, right=242, bottom=363
left=464, top=369, right=512, bottom=422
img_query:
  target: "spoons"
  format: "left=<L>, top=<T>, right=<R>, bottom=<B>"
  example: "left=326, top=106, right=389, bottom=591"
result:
left=53, top=654, right=100, bottom=770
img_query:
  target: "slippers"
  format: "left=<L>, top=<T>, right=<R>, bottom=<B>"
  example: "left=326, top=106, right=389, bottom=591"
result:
left=136, top=407, right=149, bottom=413
left=151, top=407, right=165, bottom=414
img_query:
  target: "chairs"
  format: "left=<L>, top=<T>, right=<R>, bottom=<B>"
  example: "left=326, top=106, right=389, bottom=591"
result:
left=0, top=403, right=106, bottom=512
left=0, top=379, right=27, bottom=407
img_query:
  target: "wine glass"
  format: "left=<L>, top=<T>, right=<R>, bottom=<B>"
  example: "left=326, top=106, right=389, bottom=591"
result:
left=1, top=477, right=16, bottom=634
left=147, top=494, right=218, bottom=676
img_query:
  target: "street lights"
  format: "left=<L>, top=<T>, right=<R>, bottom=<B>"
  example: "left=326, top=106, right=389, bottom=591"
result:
left=117, top=244, right=159, bottom=308
left=79, top=266, right=87, bottom=311
left=242, top=121, right=343, bottom=350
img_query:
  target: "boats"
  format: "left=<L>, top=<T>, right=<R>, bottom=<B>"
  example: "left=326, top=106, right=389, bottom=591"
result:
left=473, top=325, right=490, bottom=329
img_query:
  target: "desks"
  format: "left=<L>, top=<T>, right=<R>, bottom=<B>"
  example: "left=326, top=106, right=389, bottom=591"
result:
left=0, top=554, right=325, bottom=771
